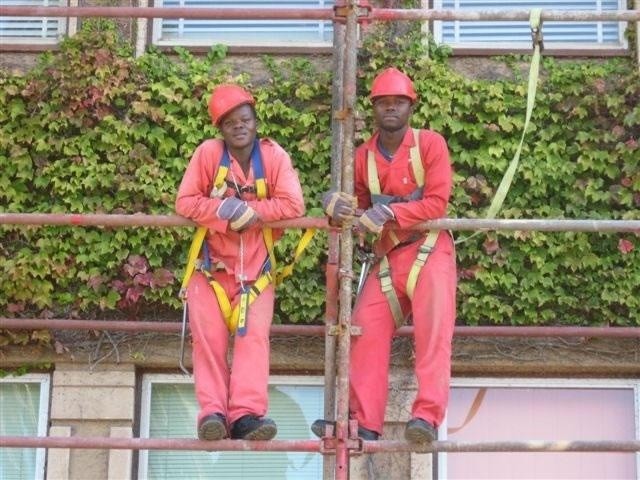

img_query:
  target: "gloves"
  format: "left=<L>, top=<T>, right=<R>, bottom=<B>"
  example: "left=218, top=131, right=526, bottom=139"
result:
left=357, top=202, right=395, bottom=235
left=322, top=191, right=359, bottom=226
left=215, top=195, right=257, bottom=232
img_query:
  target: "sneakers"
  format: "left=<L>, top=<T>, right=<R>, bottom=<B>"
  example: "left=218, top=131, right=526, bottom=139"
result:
left=197, top=413, right=228, bottom=440
left=229, top=414, right=278, bottom=440
left=311, top=417, right=379, bottom=441
left=403, top=417, right=437, bottom=444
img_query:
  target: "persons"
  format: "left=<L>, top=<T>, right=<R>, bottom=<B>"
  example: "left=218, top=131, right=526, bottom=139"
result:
left=170, top=83, right=307, bottom=445
left=312, top=65, right=459, bottom=448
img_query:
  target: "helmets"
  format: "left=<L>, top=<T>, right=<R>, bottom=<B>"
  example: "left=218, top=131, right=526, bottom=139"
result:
left=369, top=67, right=417, bottom=104
left=207, top=83, right=256, bottom=127
